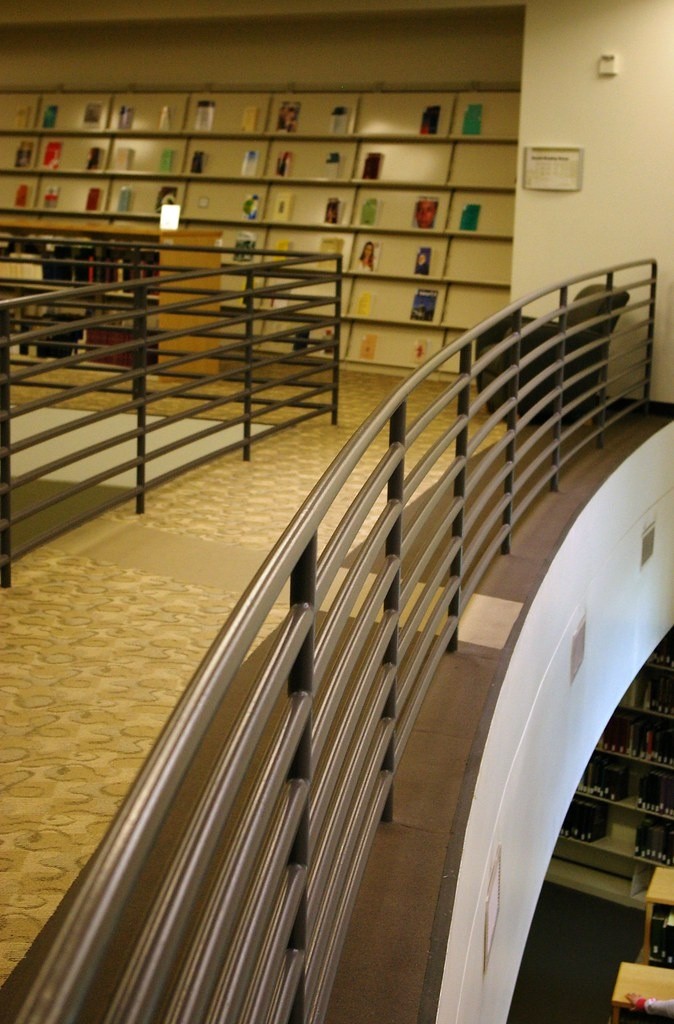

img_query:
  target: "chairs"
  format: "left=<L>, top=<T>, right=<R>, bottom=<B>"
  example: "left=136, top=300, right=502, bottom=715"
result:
left=475, top=284, right=632, bottom=427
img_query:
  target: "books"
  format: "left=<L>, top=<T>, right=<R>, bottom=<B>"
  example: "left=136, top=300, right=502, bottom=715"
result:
left=410, top=196, right=440, bottom=229
left=86, top=147, right=104, bottom=168
left=0, top=232, right=160, bottom=365
left=560, top=631, right=674, bottom=971
left=155, top=186, right=177, bottom=213
left=319, top=238, right=438, bottom=364
left=190, top=101, right=301, bottom=176
left=117, top=148, right=131, bottom=170
left=160, top=107, right=172, bottom=130
left=463, top=103, right=483, bottom=136
left=118, top=107, right=133, bottom=128
left=15, top=105, right=63, bottom=210
left=83, top=103, right=103, bottom=128
left=460, top=204, right=481, bottom=230
left=159, top=149, right=173, bottom=171
left=324, top=106, right=384, bottom=225
left=86, top=188, right=102, bottom=210
left=118, top=187, right=132, bottom=212
left=233, top=191, right=295, bottom=337
left=421, top=105, right=441, bottom=134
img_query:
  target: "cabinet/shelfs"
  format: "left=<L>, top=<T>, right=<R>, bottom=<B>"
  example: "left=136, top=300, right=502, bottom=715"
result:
left=0, top=87, right=520, bottom=378
left=553, top=626, right=674, bottom=1024
left=0, top=216, right=223, bottom=381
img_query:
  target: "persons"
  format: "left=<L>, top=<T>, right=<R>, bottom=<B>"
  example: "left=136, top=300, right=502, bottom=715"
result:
left=415, top=201, right=438, bottom=228
left=626, top=994, right=674, bottom=1019
left=358, top=242, right=375, bottom=270
